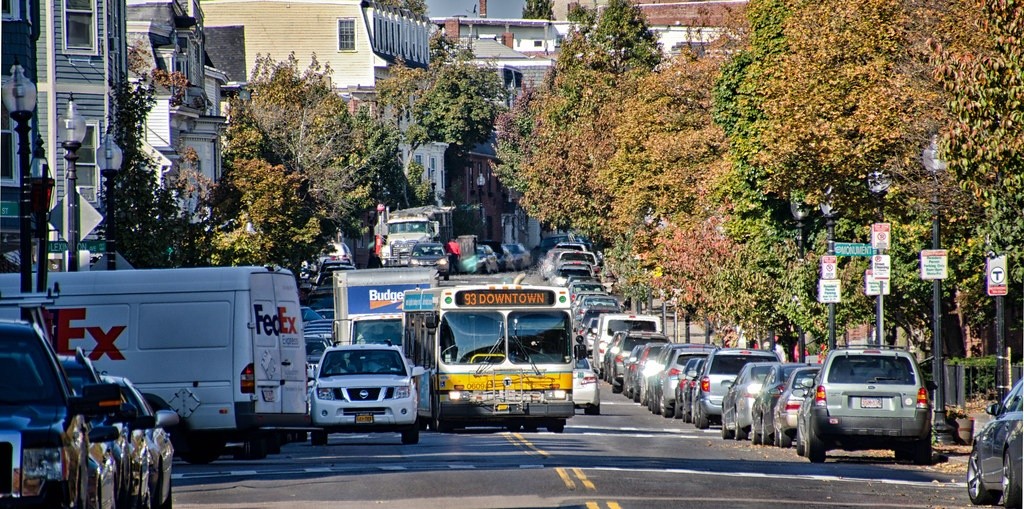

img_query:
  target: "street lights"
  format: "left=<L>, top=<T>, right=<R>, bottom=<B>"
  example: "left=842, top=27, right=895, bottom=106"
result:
left=819, top=183, right=842, bottom=352
left=1, top=65, right=37, bottom=323
left=59, top=90, right=87, bottom=273
left=868, top=168, right=893, bottom=348
left=96, top=128, right=123, bottom=271
left=476, top=173, right=486, bottom=241
left=791, top=197, right=812, bottom=365
left=923, top=134, right=959, bottom=447
left=29, top=133, right=56, bottom=293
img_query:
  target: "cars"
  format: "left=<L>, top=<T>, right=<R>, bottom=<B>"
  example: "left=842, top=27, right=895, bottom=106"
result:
left=2, top=203, right=939, bottom=508
left=966, top=377, right=1023, bottom=509
